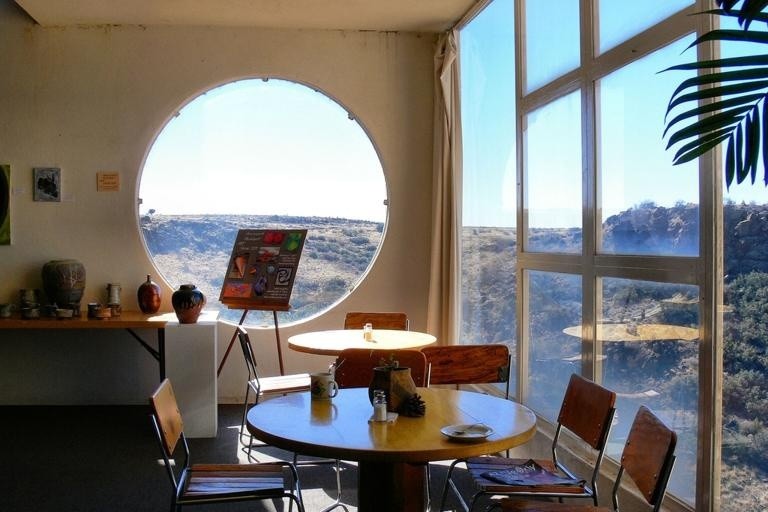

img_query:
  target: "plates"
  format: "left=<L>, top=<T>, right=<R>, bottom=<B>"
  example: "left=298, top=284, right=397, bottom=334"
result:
left=438, top=423, right=497, bottom=441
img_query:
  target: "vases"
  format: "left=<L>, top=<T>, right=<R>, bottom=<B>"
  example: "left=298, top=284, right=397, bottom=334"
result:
left=137, top=273, right=160, bottom=313
left=41, top=260, right=86, bottom=306
left=171, top=284, right=205, bottom=324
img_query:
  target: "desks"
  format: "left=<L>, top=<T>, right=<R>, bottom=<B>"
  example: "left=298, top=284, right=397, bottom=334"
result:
left=3, top=311, right=170, bottom=432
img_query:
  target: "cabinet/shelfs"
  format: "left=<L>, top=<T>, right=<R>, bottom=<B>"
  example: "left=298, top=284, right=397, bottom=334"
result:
left=164, top=311, right=223, bottom=440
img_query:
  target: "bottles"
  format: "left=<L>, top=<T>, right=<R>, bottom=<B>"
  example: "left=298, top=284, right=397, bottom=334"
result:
left=372, top=390, right=387, bottom=423
left=363, top=322, right=373, bottom=341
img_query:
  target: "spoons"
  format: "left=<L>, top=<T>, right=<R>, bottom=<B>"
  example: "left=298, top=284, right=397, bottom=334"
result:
left=454, top=422, right=483, bottom=434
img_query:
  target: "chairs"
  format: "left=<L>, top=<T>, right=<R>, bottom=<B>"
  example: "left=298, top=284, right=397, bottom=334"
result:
left=236, top=324, right=334, bottom=465
left=442, top=374, right=678, bottom=512
left=325, top=312, right=512, bottom=512
left=147, top=380, right=305, bottom=512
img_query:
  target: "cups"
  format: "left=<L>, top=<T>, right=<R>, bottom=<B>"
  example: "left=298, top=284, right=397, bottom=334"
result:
left=309, top=372, right=338, bottom=402
left=87, top=302, right=104, bottom=319
left=0, top=303, right=17, bottom=318
